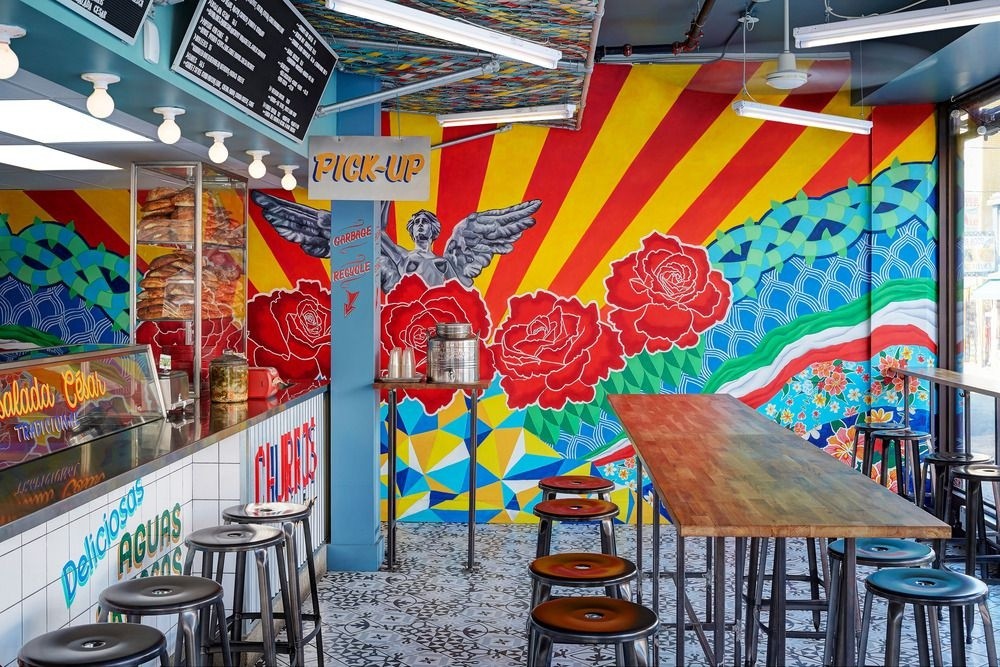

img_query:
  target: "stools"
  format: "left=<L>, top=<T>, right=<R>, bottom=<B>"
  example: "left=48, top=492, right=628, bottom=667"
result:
left=746, top=421, right=1000, bottom=667
left=17, top=501, right=324, bottom=666
left=527, top=476, right=660, bottom=667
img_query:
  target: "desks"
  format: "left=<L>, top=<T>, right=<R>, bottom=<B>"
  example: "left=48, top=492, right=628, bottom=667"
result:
left=606, top=394, right=951, bottom=667
left=372, top=378, right=491, bottom=572
left=889, top=365, right=1000, bottom=583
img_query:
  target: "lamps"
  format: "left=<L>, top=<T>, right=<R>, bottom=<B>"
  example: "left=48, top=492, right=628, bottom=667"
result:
left=436, top=104, right=576, bottom=128
left=732, top=0, right=874, bottom=135
left=793, top=0, right=1000, bottom=49
left=325, top=0, right=563, bottom=71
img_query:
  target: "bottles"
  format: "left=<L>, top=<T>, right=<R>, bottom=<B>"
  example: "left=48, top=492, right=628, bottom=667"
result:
left=209, top=350, right=249, bottom=403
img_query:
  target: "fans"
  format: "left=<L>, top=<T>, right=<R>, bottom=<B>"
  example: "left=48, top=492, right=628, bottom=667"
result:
left=651, top=0, right=938, bottom=96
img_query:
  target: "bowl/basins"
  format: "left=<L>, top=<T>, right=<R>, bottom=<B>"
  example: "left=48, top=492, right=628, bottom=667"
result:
left=436, top=323, right=472, bottom=339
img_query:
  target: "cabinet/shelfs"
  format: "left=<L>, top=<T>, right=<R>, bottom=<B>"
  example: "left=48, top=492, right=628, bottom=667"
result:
left=130, top=161, right=249, bottom=397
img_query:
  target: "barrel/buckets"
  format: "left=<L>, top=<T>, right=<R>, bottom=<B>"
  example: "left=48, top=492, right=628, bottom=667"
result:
left=427, top=337, right=480, bottom=385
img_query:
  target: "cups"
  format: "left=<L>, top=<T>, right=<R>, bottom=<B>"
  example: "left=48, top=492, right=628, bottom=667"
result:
left=387, top=347, right=416, bottom=378
